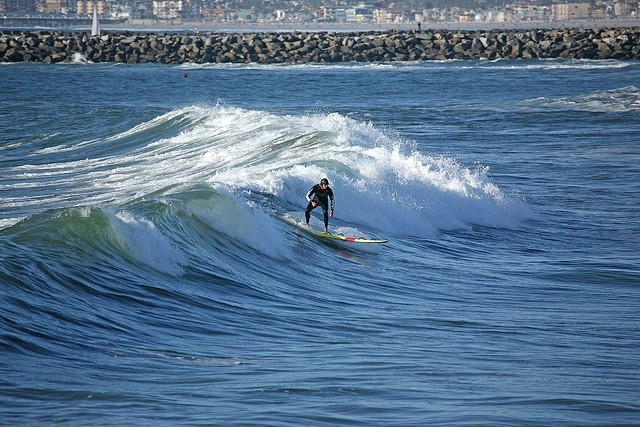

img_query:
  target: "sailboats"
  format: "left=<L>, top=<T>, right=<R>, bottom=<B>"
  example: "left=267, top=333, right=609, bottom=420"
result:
left=91, top=4, right=100, bottom=35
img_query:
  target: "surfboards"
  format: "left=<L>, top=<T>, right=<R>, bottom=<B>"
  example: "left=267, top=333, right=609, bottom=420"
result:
left=316, top=231, right=388, bottom=243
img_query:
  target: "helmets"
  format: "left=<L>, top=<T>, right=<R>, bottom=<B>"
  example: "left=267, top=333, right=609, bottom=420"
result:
left=321, top=178, right=329, bottom=184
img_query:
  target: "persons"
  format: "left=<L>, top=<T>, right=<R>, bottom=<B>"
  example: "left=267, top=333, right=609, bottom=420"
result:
left=304, top=178, right=335, bottom=232
